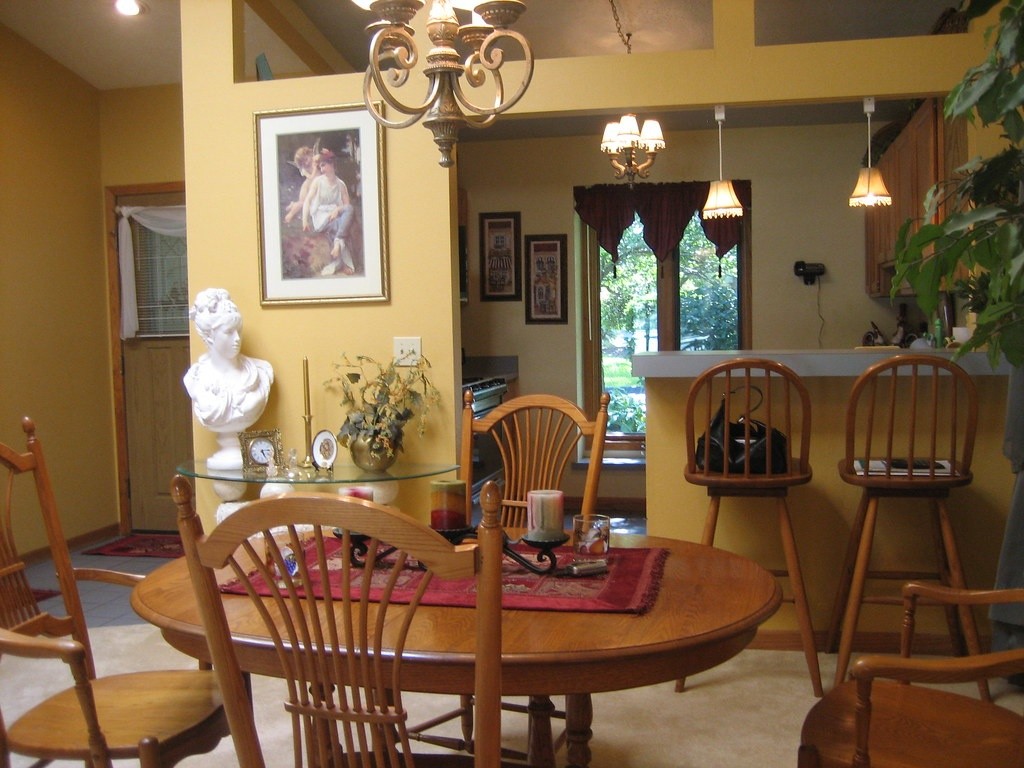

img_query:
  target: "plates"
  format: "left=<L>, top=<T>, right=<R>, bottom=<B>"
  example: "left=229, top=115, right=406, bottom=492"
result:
left=313, top=430, right=338, bottom=469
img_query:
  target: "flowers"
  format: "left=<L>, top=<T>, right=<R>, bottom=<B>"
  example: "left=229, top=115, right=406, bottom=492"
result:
left=324, top=349, right=437, bottom=459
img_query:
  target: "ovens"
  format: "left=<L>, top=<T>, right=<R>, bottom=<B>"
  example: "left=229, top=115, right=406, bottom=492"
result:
left=462, top=376, right=511, bottom=504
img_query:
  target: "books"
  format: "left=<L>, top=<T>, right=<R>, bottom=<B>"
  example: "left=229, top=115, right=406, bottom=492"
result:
left=854, top=454, right=953, bottom=477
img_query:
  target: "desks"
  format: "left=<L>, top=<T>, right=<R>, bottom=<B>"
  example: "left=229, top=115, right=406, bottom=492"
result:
left=131, top=531, right=785, bottom=767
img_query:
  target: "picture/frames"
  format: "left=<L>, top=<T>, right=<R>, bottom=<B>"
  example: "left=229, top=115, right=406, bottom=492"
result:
left=525, top=234, right=569, bottom=323
left=479, top=212, right=522, bottom=302
left=253, top=102, right=391, bottom=305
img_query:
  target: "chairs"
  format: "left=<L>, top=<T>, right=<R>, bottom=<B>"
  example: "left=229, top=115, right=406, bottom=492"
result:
left=827, top=352, right=992, bottom=705
left=464, top=389, right=611, bottom=535
left=169, top=472, right=501, bottom=768
left=0, top=415, right=250, bottom=767
left=674, top=357, right=825, bottom=698
left=800, top=587, right=1024, bottom=768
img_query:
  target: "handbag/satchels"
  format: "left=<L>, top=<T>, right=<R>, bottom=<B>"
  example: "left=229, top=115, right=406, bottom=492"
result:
left=694, top=385, right=787, bottom=475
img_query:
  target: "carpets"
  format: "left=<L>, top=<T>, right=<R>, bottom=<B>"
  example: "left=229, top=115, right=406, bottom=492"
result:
left=83, top=531, right=186, bottom=558
left=22, top=588, right=61, bottom=603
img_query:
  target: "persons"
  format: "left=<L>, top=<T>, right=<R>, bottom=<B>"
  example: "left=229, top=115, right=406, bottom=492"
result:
left=184, top=289, right=274, bottom=436
left=301, top=148, right=355, bottom=275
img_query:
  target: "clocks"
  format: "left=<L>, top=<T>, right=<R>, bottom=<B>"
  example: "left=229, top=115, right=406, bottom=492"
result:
left=238, top=429, right=287, bottom=476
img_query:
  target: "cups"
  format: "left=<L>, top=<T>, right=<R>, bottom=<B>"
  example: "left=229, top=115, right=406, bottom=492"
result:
left=909, top=333, right=934, bottom=349
left=944, top=336, right=964, bottom=352
left=572, top=513, right=610, bottom=567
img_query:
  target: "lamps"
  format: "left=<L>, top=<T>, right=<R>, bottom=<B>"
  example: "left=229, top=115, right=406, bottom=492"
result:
left=848, top=96, right=892, bottom=206
left=702, top=105, right=743, bottom=219
left=600, top=114, right=666, bottom=188
left=350, top=0, right=533, bottom=166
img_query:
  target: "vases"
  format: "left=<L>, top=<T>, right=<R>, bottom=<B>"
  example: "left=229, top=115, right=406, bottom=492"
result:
left=350, top=430, right=398, bottom=471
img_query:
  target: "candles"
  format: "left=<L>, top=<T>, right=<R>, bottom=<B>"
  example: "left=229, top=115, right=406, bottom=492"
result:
left=336, top=487, right=374, bottom=532
left=527, top=490, right=565, bottom=543
left=429, top=479, right=466, bottom=533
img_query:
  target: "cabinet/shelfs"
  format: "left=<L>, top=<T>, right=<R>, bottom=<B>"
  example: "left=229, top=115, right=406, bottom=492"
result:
left=864, top=99, right=955, bottom=298
